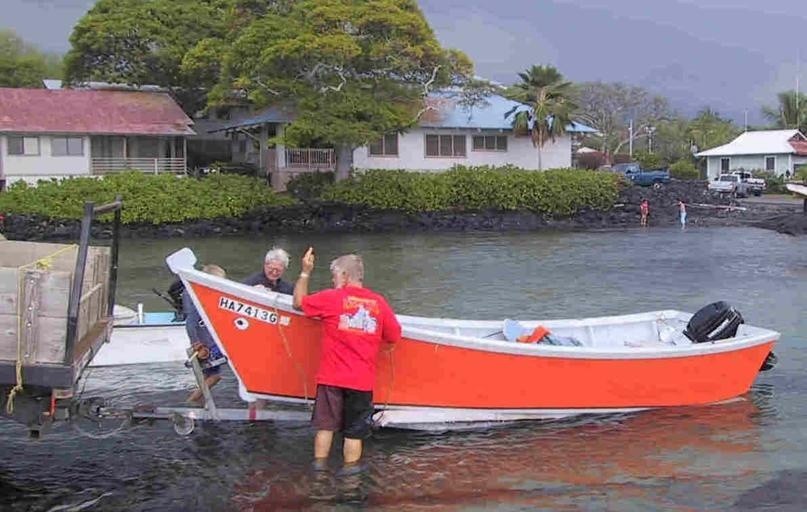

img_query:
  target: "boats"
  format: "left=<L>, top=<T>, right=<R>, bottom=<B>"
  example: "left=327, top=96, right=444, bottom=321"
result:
left=86, top=311, right=190, bottom=367
left=165, top=247, right=781, bottom=428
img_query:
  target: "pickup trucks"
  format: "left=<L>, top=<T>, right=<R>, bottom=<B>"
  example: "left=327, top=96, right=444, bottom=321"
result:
left=708, top=171, right=766, bottom=198
left=612, top=163, right=669, bottom=187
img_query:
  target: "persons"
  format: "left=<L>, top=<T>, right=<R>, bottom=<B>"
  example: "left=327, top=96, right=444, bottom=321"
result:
left=292, top=247, right=401, bottom=460
left=640, top=199, right=650, bottom=225
left=677, top=201, right=687, bottom=228
left=785, top=170, right=791, bottom=181
left=246, top=246, right=293, bottom=295
left=181, top=265, right=228, bottom=402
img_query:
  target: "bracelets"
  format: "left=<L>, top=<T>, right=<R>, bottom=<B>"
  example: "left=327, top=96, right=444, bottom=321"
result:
left=301, top=272, right=310, bottom=279
